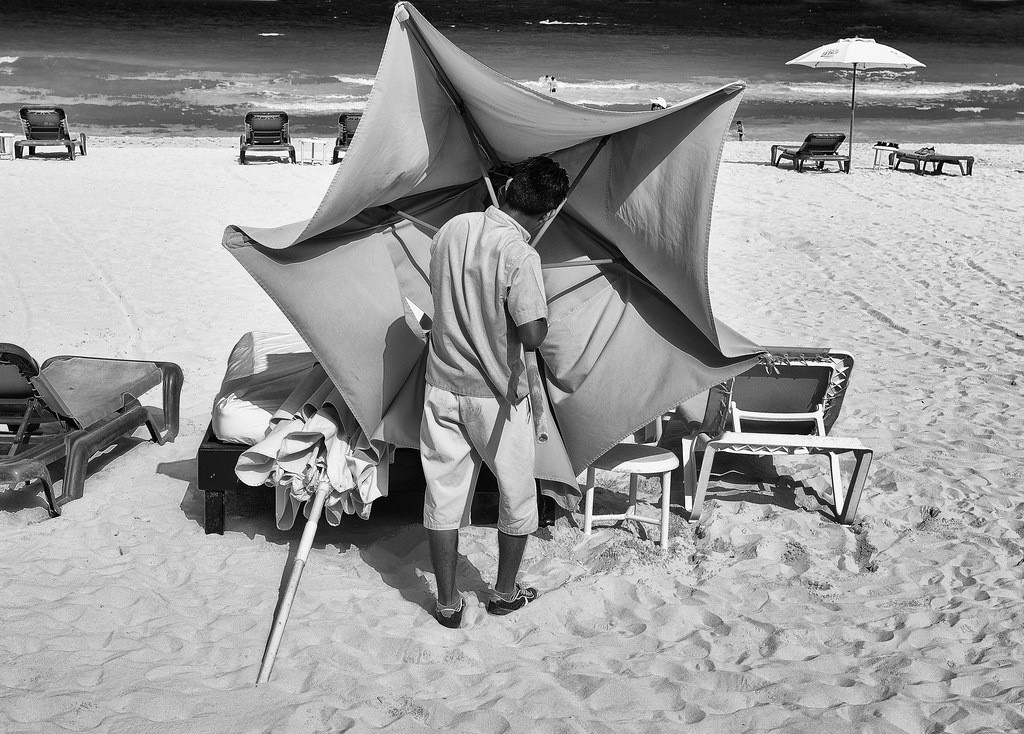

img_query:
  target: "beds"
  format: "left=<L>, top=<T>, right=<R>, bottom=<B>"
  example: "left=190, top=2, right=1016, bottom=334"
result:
left=196, top=329, right=557, bottom=535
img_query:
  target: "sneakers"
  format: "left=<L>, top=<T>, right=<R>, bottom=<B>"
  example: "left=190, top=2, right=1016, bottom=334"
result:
left=487, top=581, right=538, bottom=615
left=436, top=591, right=465, bottom=628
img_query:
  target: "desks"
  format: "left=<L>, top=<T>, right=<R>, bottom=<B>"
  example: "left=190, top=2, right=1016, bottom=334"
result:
left=873, top=144, right=899, bottom=172
left=0, top=130, right=15, bottom=160
left=300, top=138, right=328, bottom=166
left=582, top=443, right=680, bottom=550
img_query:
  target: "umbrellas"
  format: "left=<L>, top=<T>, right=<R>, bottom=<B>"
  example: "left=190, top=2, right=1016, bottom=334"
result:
left=223, top=0, right=775, bottom=686
left=784, top=35, right=926, bottom=174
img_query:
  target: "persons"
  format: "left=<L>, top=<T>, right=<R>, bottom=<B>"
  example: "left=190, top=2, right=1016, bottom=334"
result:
left=544, top=74, right=556, bottom=95
left=420, top=156, right=570, bottom=629
left=736, top=120, right=744, bottom=141
left=649, top=98, right=667, bottom=110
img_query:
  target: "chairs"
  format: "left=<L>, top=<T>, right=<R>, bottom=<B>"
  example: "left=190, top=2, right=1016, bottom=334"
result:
left=888, top=148, right=975, bottom=175
left=771, top=132, right=849, bottom=173
left=14, top=104, right=88, bottom=161
left=333, top=111, right=364, bottom=165
left=240, top=111, right=297, bottom=165
left=645, top=348, right=874, bottom=524
left=0, top=342, right=184, bottom=518
